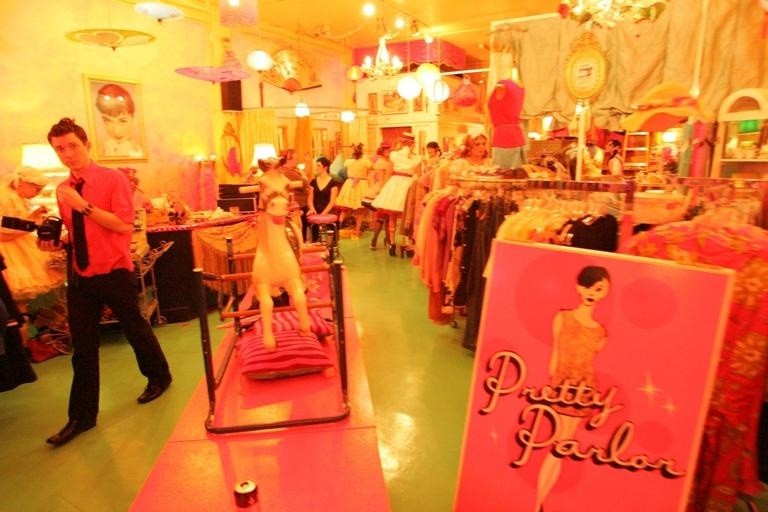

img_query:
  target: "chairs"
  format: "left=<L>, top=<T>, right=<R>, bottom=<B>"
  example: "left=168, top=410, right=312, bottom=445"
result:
left=451, top=178, right=627, bottom=203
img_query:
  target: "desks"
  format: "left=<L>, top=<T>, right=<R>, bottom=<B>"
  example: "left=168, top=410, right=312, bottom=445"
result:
left=170, top=317, right=375, bottom=443
left=238, top=268, right=355, bottom=318
left=145, top=215, right=256, bottom=323
left=130, top=427, right=390, bottom=510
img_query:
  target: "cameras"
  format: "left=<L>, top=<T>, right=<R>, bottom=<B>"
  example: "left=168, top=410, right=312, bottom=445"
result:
left=1, top=216, right=64, bottom=246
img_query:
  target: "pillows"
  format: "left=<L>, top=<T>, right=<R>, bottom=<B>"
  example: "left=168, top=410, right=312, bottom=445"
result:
left=230, top=328, right=336, bottom=381
left=253, top=306, right=337, bottom=338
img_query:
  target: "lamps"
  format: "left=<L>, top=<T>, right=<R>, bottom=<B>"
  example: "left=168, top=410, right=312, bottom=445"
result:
left=246, top=48, right=273, bottom=108
left=294, top=102, right=310, bottom=119
left=541, top=114, right=553, bottom=133
left=410, top=17, right=422, bottom=38
left=251, top=143, right=278, bottom=167
left=340, top=110, right=356, bottom=123
left=360, top=32, right=404, bottom=78
left=20, top=143, right=64, bottom=169
left=396, top=37, right=450, bottom=105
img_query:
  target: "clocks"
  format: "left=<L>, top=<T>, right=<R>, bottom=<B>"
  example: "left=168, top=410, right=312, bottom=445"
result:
left=562, top=31, right=612, bottom=108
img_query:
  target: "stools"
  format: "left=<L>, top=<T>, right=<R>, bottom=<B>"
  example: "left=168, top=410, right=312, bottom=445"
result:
left=305, top=214, right=339, bottom=244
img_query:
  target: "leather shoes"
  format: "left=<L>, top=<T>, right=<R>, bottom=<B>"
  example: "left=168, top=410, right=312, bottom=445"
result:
left=46, top=419, right=97, bottom=445
left=137, top=374, right=172, bottom=403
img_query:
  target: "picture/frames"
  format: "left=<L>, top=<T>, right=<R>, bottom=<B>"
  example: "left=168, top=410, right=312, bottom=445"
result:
left=82, top=72, right=149, bottom=165
left=452, top=236, right=739, bottom=512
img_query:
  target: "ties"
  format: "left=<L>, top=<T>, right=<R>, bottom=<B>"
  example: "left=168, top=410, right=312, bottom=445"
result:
left=72, top=179, right=88, bottom=270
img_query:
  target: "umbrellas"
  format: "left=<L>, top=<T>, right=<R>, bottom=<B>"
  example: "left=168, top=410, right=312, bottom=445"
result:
left=66, top=9, right=156, bottom=52
left=174, top=41, right=251, bottom=86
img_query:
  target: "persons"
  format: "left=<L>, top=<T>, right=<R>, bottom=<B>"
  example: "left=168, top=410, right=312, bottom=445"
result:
left=585, top=140, right=604, bottom=168
left=533, top=266, right=610, bottom=512
left=661, top=145, right=679, bottom=174
left=94, top=83, right=143, bottom=157
left=336, top=136, right=443, bottom=258
left=35, top=117, right=173, bottom=445
left=491, top=66, right=526, bottom=170
left=1, top=166, right=67, bottom=347
left=302, top=157, right=338, bottom=243
left=603, top=137, right=626, bottom=176
left=450, top=132, right=494, bottom=180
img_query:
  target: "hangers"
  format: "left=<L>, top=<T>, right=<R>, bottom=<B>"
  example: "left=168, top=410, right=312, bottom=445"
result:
left=663, top=174, right=768, bottom=187
left=446, top=176, right=636, bottom=329
